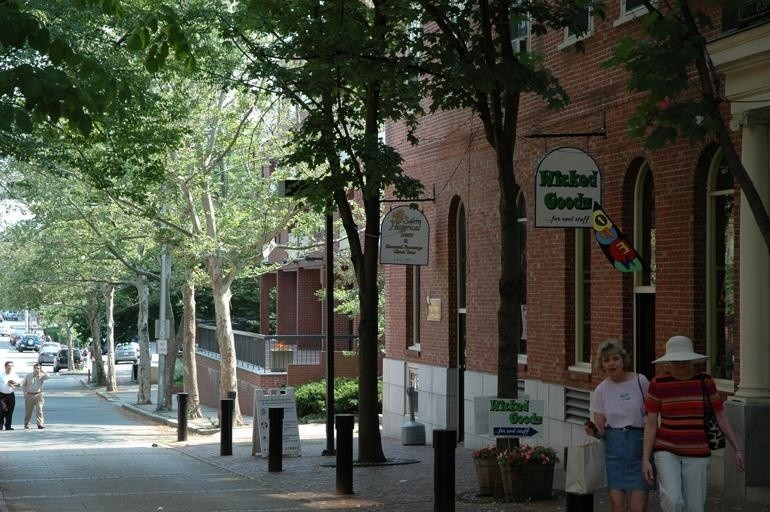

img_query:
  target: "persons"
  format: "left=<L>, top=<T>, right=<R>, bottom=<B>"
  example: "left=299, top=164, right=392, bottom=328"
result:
left=583, top=338, right=655, bottom=511
left=641, top=335, right=746, bottom=512
left=23, top=362, right=52, bottom=428
left=0, top=361, right=23, bottom=429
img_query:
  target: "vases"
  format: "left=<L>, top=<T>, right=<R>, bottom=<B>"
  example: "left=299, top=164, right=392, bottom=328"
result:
left=500, top=464, right=554, bottom=503
left=474, top=458, right=499, bottom=497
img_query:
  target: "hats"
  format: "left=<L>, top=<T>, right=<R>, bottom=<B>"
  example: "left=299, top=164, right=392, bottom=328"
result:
left=650, top=336, right=711, bottom=365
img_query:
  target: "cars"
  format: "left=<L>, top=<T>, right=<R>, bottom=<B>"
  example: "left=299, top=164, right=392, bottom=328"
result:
left=0, top=309, right=87, bottom=373
left=89, top=340, right=140, bottom=363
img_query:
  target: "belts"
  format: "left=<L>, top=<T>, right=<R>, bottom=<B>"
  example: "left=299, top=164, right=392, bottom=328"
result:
left=27, top=392, right=42, bottom=395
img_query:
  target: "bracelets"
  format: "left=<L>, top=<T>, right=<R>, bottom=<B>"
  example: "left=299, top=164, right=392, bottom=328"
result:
left=733, top=449, right=744, bottom=452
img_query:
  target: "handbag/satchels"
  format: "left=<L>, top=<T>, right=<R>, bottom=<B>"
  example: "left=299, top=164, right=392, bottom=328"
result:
left=704, top=409, right=726, bottom=451
left=565, top=434, right=609, bottom=495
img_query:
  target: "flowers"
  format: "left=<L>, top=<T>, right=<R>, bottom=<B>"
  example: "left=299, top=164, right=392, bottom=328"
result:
left=497, top=444, right=559, bottom=465
left=470, top=445, right=498, bottom=460
